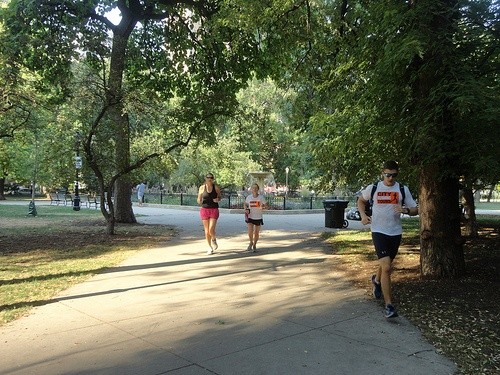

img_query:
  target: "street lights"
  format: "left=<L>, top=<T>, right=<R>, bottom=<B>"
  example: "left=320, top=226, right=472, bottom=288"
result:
left=72, top=131, right=80, bottom=211
left=285, top=167, right=289, bottom=199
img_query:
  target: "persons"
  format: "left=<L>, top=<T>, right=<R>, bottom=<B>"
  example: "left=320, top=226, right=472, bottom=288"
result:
left=357, top=160, right=418, bottom=318
left=244, top=184, right=269, bottom=253
left=136, top=183, right=147, bottom=207
left=197, top=173, right=221, bottom=255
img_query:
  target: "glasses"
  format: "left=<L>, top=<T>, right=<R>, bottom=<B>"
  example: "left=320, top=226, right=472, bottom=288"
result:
left=206, top=177, right=213, bottom=179
left=383, top=173, right=398, bottom=178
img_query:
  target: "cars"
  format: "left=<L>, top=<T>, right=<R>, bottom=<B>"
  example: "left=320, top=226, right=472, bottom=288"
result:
left=18, top=188, right=35, bottom=196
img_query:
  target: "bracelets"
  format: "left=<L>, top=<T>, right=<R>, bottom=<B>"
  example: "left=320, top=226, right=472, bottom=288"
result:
left=403, top=208, right=410, bottom=214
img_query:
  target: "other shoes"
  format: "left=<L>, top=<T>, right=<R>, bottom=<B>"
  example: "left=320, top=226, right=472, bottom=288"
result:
left=252, top=245, right=257, bottom=252
left=212, top=240, right=218, bottom=250
left=246, top=243, right=253, bottom=250
left=137, top=202, right=143, bottom=206
left=207, top=247, right=213, bottom=255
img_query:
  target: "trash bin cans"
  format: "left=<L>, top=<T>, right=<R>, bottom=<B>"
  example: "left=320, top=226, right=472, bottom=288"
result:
left=322, top=199, right=349, bottom=229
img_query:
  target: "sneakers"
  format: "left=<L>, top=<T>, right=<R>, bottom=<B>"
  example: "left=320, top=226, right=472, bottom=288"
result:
left=371, top=274, right=382, bottom=300
left=385, top=303, right=398, bottom=318
